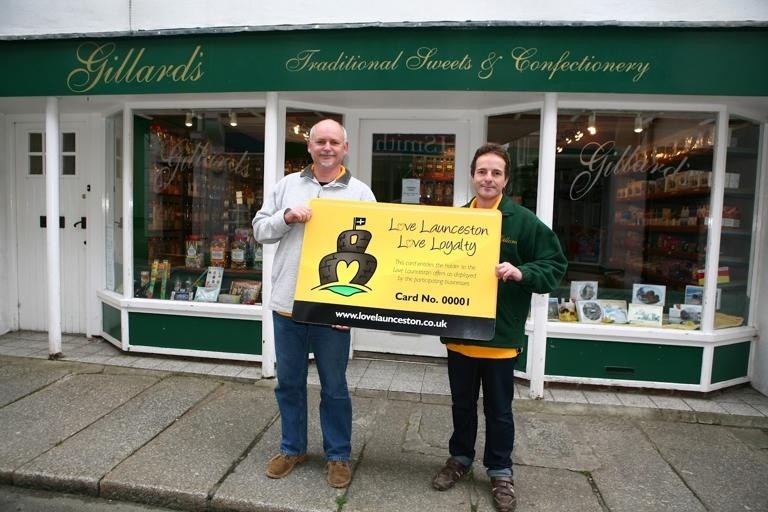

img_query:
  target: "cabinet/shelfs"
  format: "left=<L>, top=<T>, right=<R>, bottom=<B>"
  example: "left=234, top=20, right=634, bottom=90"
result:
left=607, top=145, right=759, bottom=293
left=151, top=158, right=262, bottom=268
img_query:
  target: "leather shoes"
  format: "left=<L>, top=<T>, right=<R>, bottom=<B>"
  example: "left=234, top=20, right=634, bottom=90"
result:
left=325, top=458, right=352, bottom=488
left=264, top=451, right=306, bottom=480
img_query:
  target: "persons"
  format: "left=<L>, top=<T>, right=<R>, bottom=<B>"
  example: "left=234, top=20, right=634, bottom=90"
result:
left=251, top=118, right=377, bottom=488
left=432, top=142, right=569, bottom=512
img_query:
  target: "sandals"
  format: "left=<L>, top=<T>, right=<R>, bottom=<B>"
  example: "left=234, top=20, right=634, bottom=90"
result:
left=431, top=458, right=472, bottom=492
left=488, top=470, right=516, bottom=512
